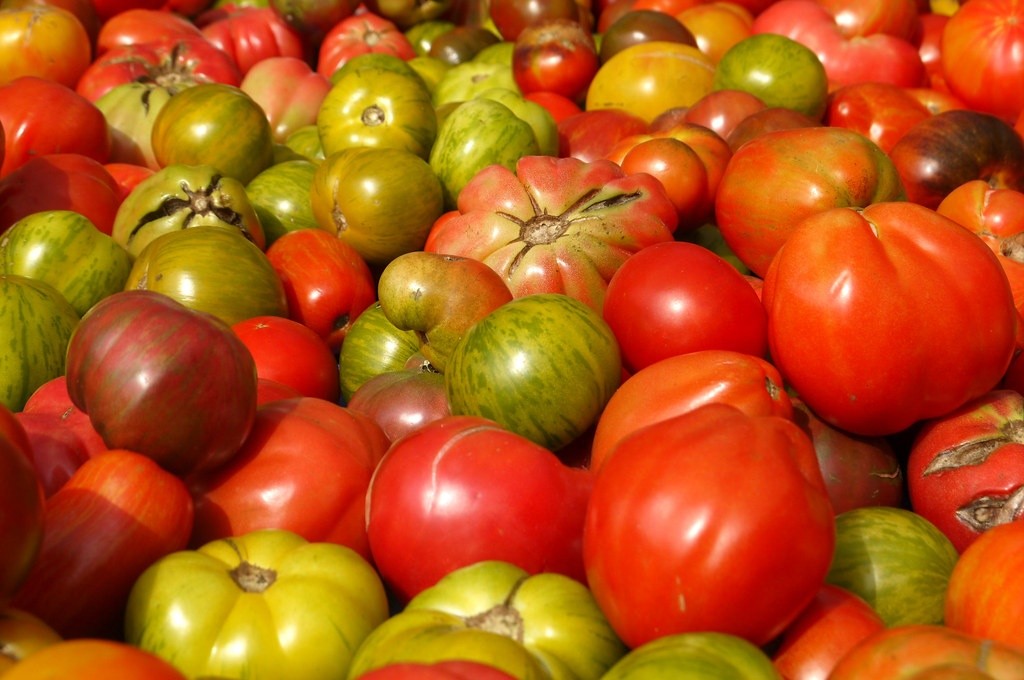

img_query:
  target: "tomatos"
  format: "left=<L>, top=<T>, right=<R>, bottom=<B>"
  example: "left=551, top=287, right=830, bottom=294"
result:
left=0, top=0, right=1024, bottom=680
